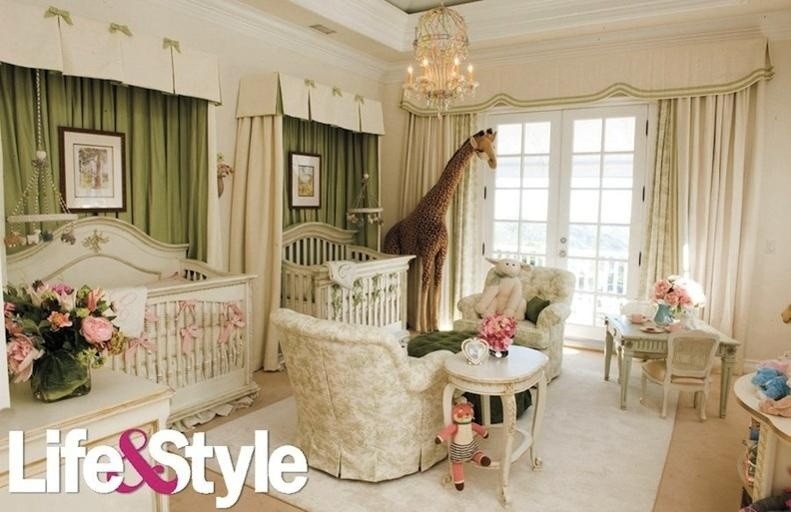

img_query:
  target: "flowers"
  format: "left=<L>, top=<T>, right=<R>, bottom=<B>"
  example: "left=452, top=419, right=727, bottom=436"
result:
left=648, top=274, right=705, bottom=308
left=478, top=311, right=518, bottom=352
left=2, top=279, right=125, bottom=392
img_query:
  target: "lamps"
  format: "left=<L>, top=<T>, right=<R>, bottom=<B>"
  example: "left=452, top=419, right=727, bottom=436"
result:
left=402, top=2, right=480, bottom=121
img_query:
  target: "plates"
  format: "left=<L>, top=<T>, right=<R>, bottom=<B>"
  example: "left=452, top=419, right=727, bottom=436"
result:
left=630, top=320, right=647, bottom=324
left=665, top=327, right=672, bottom=332
left=640, top=327, right=665, bottom=333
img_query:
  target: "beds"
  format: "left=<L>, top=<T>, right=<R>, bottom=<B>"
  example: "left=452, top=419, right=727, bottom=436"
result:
left=281, top=221, right=417, bottom=331
left=7, top=216, right=261, bottom=432
left=409, top=330, right=532, bottom=426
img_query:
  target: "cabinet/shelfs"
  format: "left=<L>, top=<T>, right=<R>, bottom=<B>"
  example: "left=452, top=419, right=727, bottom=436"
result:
left=733, top=372, right=791, bottom=512
left=0, top=368, right=176, bottom=512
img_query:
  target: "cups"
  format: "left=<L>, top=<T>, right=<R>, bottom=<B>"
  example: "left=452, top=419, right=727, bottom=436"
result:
left=631, top=314, right=641, bottom=322
left=670, top=323, right=681, bottom=331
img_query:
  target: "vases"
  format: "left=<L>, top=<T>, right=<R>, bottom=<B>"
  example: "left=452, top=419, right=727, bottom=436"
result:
left=654, top=304, right=673, bottom=326
left=30, top=349, right=91, bottom=404
left=489, top=348, right=508, bottom=358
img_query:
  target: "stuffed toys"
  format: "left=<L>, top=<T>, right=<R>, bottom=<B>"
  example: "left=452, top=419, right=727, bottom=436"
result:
left=750, top=359, right=791, bottom=418
left=475, top=255, right=534, bottom=322
left=434, top=400, right=491, bottom=492
left=4, top=228, right=76, bottom=249
left=383, top=127, right=498, bottom=335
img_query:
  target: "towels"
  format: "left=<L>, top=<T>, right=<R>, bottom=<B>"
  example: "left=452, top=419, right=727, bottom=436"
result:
left=108, top=285, right=147, bottom=339
left=321, top=259, right=358, bottom=291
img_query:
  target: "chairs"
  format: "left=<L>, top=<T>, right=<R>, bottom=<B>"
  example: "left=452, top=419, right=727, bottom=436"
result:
left=454, top=265, right=575, bottom=388
left=270, top=307, right=456, bottom=483
left=640, top=329, right=720, bottom=423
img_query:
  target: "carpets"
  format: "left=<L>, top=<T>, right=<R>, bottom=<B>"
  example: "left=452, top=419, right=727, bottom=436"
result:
left=167, top=352, right=680, bottom=512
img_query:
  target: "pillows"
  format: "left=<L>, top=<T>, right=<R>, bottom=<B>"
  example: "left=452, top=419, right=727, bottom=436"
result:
left=524, top=295, right=550, bottom=324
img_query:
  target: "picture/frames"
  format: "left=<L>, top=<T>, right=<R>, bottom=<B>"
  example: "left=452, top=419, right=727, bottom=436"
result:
left=288, top=151, right=322, bottom=209
left=59, top=126, right=126, bottom=212
